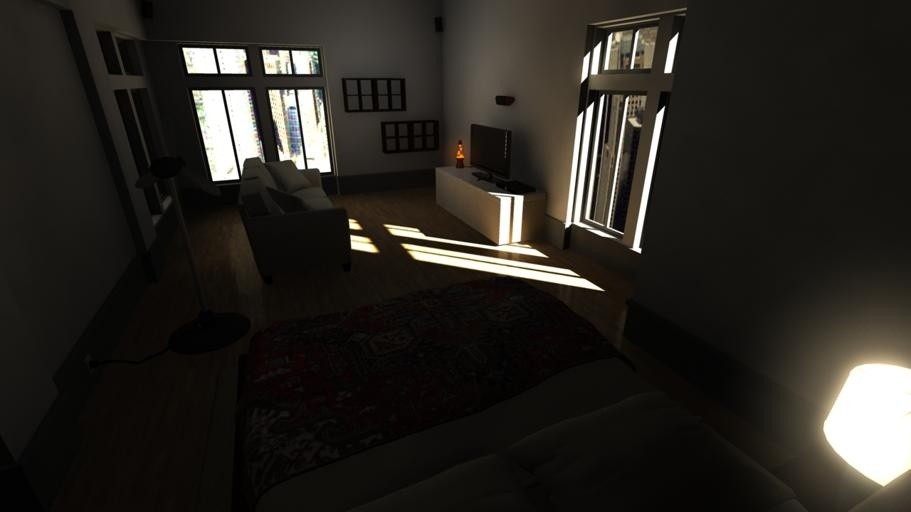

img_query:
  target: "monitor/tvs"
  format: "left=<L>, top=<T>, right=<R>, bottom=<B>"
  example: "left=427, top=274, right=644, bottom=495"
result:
left=468, top=121, right=512, bottom=185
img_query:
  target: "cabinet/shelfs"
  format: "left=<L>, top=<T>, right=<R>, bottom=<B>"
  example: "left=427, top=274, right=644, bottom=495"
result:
left=343, top=78, right=406, bottom=112
left=382, top=120, right=438, bottom=153
left=59, top=6, right=184, bottom=253
left=435, top=166, right=546, bottom=245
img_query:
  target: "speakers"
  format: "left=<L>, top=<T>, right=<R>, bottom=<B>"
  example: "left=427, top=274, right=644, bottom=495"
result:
left=495, top=95, right=516, bottom=106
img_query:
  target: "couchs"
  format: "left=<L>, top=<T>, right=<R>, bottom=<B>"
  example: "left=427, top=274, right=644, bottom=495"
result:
left=237, top=158, right=352, bottom=287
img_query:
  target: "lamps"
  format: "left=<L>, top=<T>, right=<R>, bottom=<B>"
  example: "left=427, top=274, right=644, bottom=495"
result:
left=456, top=140, right=464, bottom=168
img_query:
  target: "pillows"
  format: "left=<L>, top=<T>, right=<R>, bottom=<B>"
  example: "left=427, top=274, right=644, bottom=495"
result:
left=262, top=160, right=314, bottom=212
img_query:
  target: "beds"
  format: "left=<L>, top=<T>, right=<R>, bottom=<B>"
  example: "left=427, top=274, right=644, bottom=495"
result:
left=251, top=277, right=809, bottom=511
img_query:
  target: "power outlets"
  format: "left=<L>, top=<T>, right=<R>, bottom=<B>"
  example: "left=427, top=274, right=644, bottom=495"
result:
left=84, top=353, right=96, bottom=375
